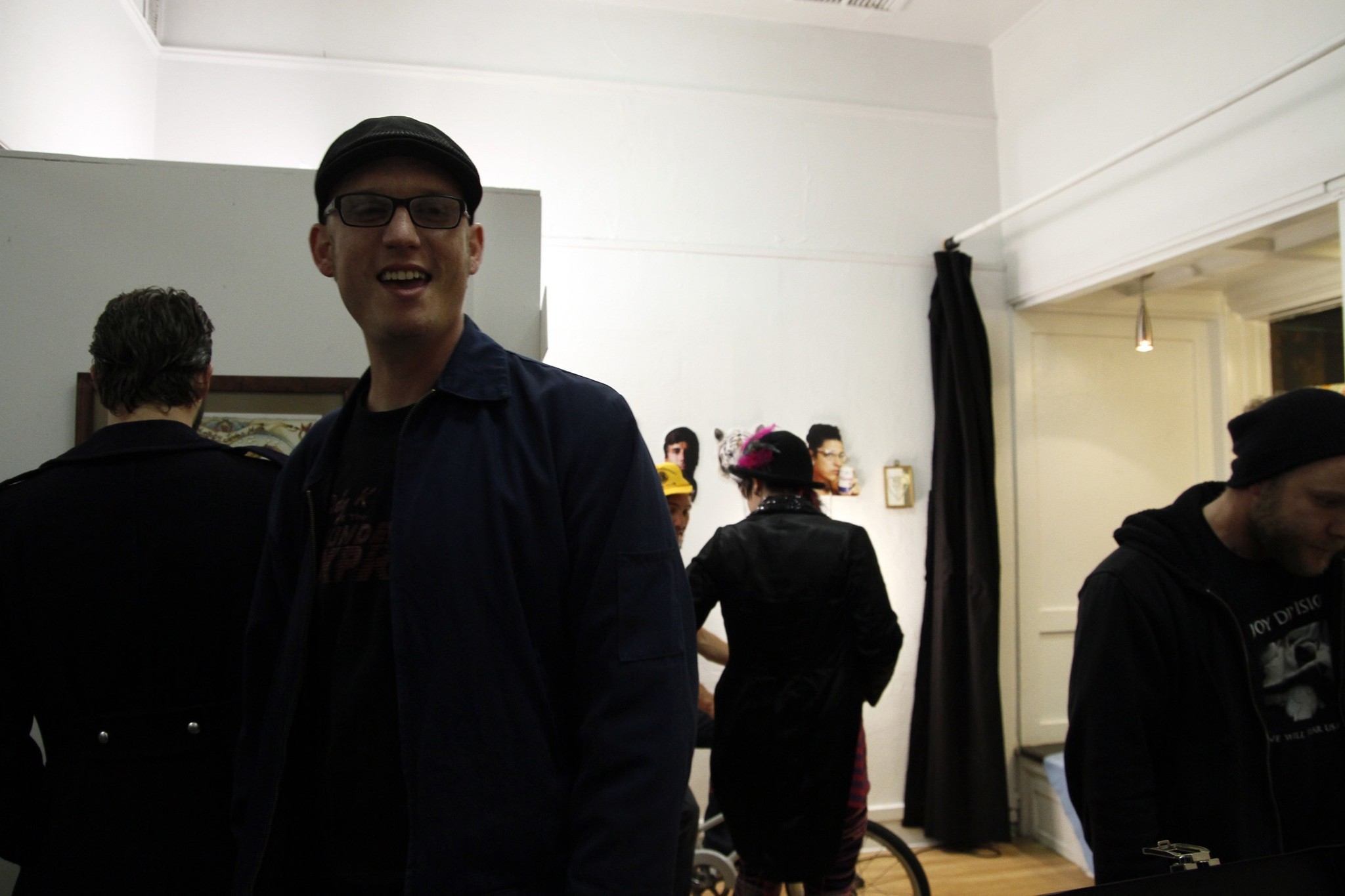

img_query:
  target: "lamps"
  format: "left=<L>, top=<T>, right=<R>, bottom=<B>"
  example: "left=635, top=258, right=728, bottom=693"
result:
left=1132, top=272, right=1157, bottom=356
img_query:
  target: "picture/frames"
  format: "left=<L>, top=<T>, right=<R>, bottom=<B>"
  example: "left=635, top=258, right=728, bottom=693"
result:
left=883, top=459, right=917, bottom=513
left=71, top=369, right=368, bottom=467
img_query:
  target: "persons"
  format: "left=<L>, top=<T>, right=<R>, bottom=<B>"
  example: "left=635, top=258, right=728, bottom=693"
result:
left=772, top=713, right=871, bottom=896
left=242, top=116, right=702, bottom=896
left=652, top=462, right=730, bottom=896
left=669, top=425, right=903, bottom=896
left=663, top=426, right=699, bottom=502
left=806, top=423, right=846, bottom=495
left=0, top=285, right=283, bottom=896
left=1060, top=388, right=1345, bottom=886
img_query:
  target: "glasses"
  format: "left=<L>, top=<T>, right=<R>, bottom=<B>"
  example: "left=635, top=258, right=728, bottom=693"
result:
left=815, top=446, right=847, bottom=464
left=323, top=191, right=473, bottom=230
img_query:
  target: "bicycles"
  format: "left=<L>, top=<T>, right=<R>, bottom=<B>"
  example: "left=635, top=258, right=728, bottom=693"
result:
left=688, top=813, right=930, bottom=896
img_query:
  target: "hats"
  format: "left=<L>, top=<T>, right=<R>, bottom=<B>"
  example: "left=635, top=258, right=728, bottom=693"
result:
left=314, top=115, right=483, bottom=210
left=654, top=463, right=693, bottom=496
left=727, top=422, right=826, bottom=490
left=1229, top=388, right=1345, bottom=492
